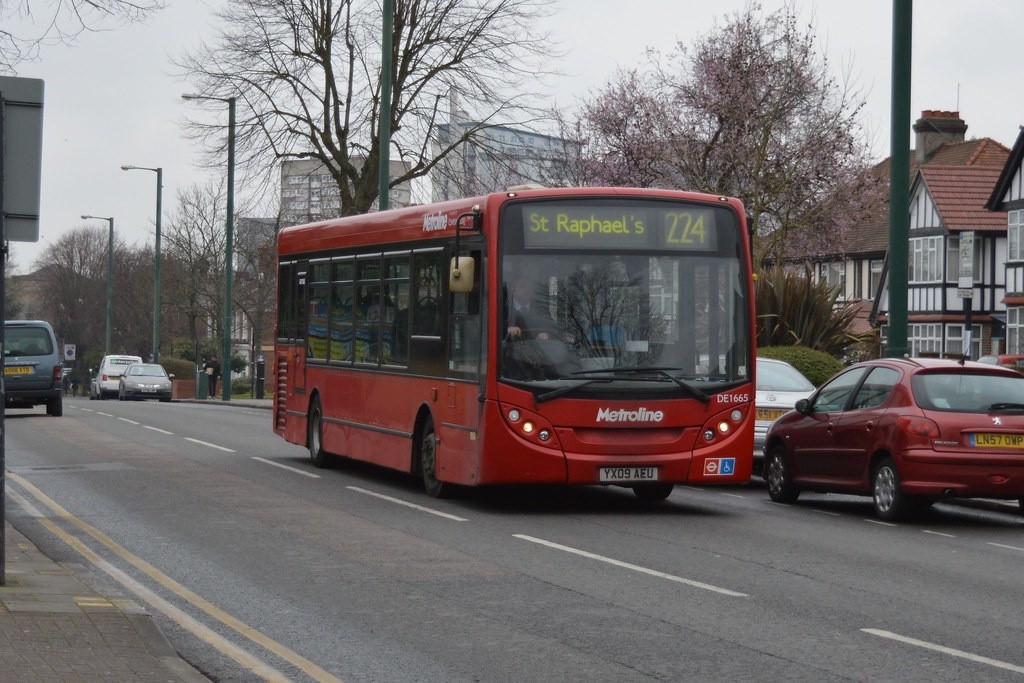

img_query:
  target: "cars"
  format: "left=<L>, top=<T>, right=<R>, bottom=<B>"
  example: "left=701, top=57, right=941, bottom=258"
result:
left=89, top=377, right=98, bottom=400
left=691, top=350, right=830, bottom=484
left=116, top=362, right=175, bottom=402
left=759, top=354, right=1024, bottom=521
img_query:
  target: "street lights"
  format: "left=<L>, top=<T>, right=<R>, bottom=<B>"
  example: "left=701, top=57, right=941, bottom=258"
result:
left=179, top=93, right=236, bottom=401
left=121, top=166, right=164, bottom=363
left=81, top=214, right=114, bottom=356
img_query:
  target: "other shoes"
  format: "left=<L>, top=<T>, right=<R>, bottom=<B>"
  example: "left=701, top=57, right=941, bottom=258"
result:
left=209, top=395, right=216, bottom=400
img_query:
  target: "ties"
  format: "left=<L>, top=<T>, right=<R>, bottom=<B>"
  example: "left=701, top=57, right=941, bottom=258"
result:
left=521, top=307, right=528, bottom=313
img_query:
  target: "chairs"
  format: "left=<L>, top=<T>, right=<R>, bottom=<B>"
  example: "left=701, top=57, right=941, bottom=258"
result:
left=586, top=325, right=631, bottom=350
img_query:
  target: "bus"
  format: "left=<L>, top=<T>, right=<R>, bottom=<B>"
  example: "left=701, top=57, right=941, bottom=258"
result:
left=270, top=185, right=759, bottom=505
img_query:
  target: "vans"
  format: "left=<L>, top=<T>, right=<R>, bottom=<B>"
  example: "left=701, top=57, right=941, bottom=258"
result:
left=93, top=353, right=144, bottom=400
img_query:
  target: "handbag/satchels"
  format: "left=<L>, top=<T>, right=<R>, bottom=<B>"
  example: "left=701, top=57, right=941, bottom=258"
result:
left=206, top=367, right=214, bottom=376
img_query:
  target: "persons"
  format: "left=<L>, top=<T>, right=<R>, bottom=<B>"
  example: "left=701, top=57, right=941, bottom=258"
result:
left=63, top=377, right=78, bottom=397
left=203, top=355, right=220, bottom=399
left=503, top=276, right=576, bottom=343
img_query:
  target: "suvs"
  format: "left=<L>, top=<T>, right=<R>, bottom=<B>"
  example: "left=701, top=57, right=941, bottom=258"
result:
left=3, top=318, right=65, bottom=417
